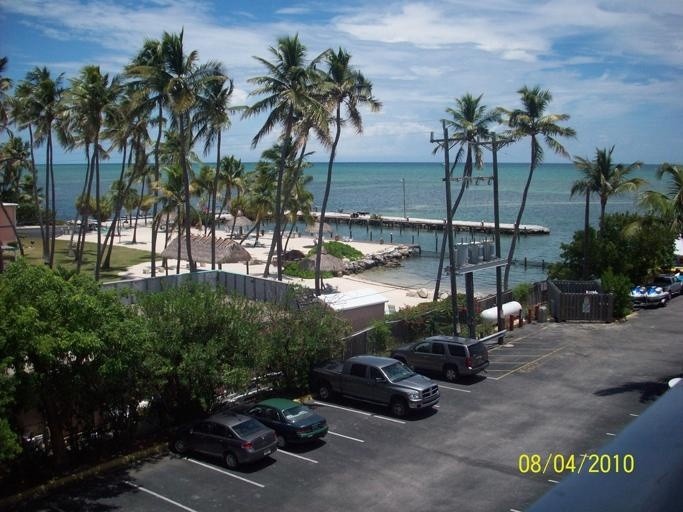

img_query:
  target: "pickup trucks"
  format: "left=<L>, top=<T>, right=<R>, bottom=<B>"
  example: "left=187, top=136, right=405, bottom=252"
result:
left=312, top=355, right=441, bottom=419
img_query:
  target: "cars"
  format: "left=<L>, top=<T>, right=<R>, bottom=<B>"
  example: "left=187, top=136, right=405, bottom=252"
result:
left=628, top=255, right=683, bottom=307
left=169, top=398, right=328, bottom=470
left=391, top=336, right=489, bottom=382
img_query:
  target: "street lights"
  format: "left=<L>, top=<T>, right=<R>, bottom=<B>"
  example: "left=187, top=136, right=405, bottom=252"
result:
left=400, top=178, right=406, bottom=218
